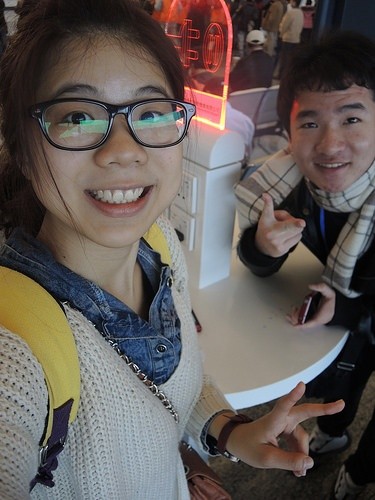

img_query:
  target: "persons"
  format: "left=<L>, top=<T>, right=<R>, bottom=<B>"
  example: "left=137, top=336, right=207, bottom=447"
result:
left=229, top=30, right=375, bottom=500
left=0, top=1, right=346, bottom=498
left=186, top=28, right=274, bottom=98
left=273, top=1, right=305, bottom=81
left=150, top=0, right=318, bottom=64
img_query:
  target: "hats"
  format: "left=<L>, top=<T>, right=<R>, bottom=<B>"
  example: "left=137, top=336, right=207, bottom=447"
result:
left=246, top=30, right=265, bottom=45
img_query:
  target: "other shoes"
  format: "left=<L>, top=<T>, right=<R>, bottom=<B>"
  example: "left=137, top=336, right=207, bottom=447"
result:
left=332, top=454, right=367, bottom=499
left=308, top=425, right=352, bottom=464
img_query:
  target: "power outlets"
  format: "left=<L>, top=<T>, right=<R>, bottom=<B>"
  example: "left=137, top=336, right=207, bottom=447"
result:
left=163, top=169, right=198, bottom=251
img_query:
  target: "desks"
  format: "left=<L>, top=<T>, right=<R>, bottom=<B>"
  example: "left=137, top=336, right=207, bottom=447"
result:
left=191, top=209, right=350, bottom=467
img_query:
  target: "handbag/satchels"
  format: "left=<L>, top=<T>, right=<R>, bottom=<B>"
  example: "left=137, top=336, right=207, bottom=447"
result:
left=177, top=440, right=235, bottom=500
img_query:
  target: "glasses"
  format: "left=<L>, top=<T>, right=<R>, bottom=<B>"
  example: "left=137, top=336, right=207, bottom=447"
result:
left=25, top=97, right=195, bottom=149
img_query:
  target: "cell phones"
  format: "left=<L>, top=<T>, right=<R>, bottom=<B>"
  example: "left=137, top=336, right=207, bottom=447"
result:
left=298, top=289, right=321, bottom=325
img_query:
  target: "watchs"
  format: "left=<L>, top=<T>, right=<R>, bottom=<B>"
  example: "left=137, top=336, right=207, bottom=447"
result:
left=213, top=411, right=255, bottom=464
